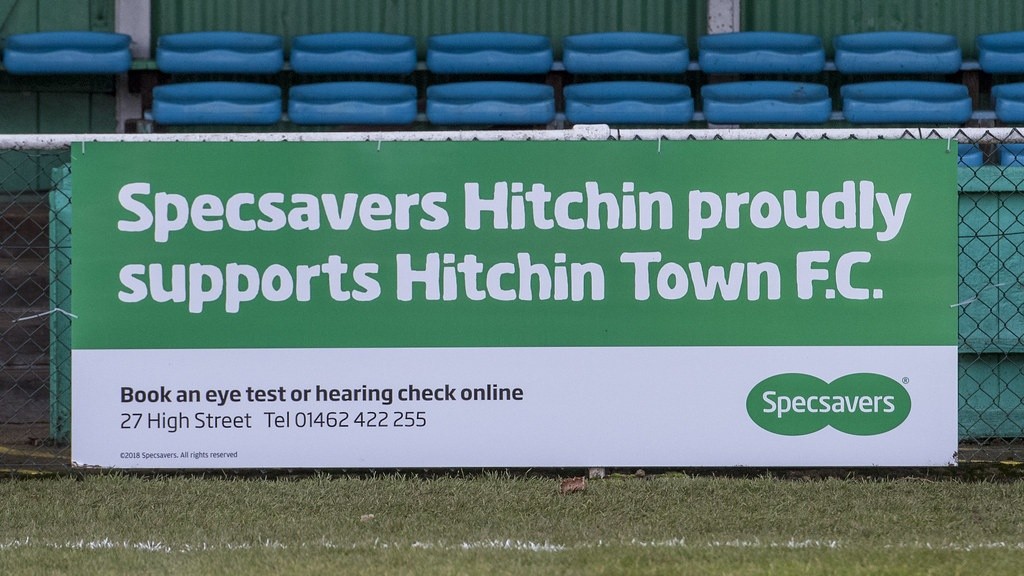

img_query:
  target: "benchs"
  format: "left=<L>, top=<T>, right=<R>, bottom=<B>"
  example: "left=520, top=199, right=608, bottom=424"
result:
left=5, top=31, right=1024, bottom=173
